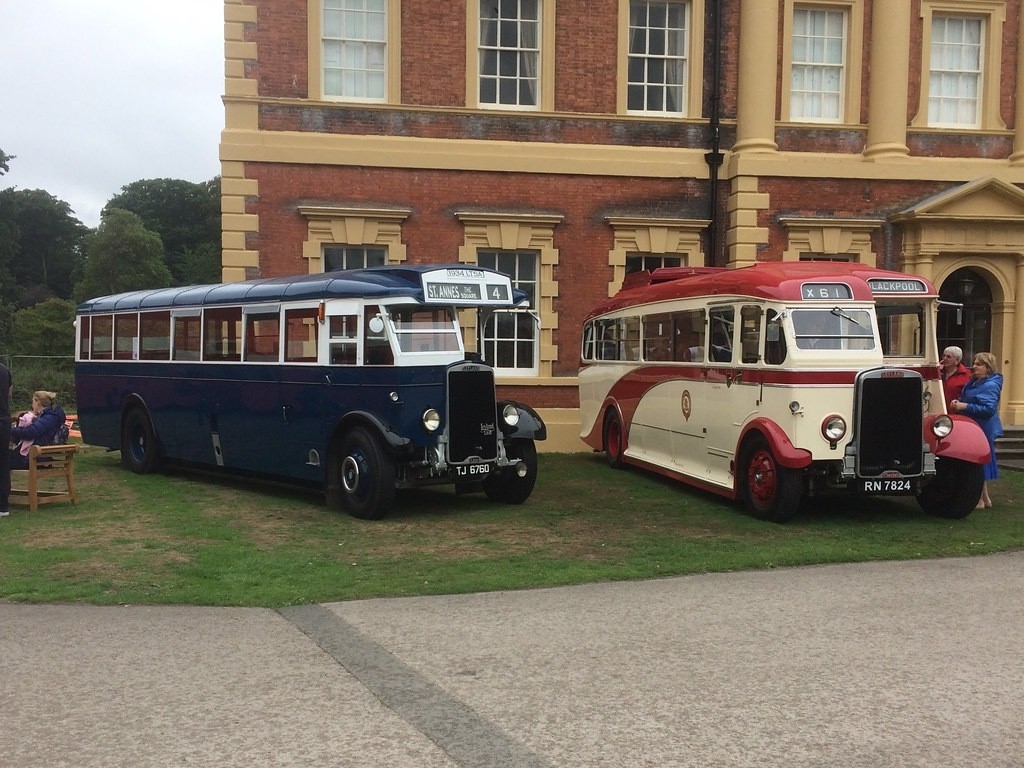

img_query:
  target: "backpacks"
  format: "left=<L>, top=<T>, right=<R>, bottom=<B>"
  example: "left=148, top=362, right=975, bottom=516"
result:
left=51, top=423, right=69, bottom=445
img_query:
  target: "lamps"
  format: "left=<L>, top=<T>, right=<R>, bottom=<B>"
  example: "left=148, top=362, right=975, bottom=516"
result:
left=959, top=269, right=976, bottom=304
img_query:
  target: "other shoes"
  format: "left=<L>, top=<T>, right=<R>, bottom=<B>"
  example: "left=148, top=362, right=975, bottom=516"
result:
left=0, top=508, right=9, bottom=516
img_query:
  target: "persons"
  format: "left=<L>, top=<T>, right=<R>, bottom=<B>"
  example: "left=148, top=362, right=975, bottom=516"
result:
left=949, top=352, right=1006, bottom=510
left=938, top=346, right=972, bottom=417
left=9, top=390, right=69, bottom=471
left=650, top=336, right=669, bottom=361
left=0, top=364, right=13, bottom=515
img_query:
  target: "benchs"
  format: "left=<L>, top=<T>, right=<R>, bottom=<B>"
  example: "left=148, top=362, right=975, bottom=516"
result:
left=8, top=443, right=80, bottom=512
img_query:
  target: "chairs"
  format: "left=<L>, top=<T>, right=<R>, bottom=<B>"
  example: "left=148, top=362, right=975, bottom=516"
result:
left=600, top=346, right=718, bottom=363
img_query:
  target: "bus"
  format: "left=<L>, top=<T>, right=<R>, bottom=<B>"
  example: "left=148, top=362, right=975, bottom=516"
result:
left=73, top=266, right=547, bottom=521
left=576, top=258, right=993, bottom=526
